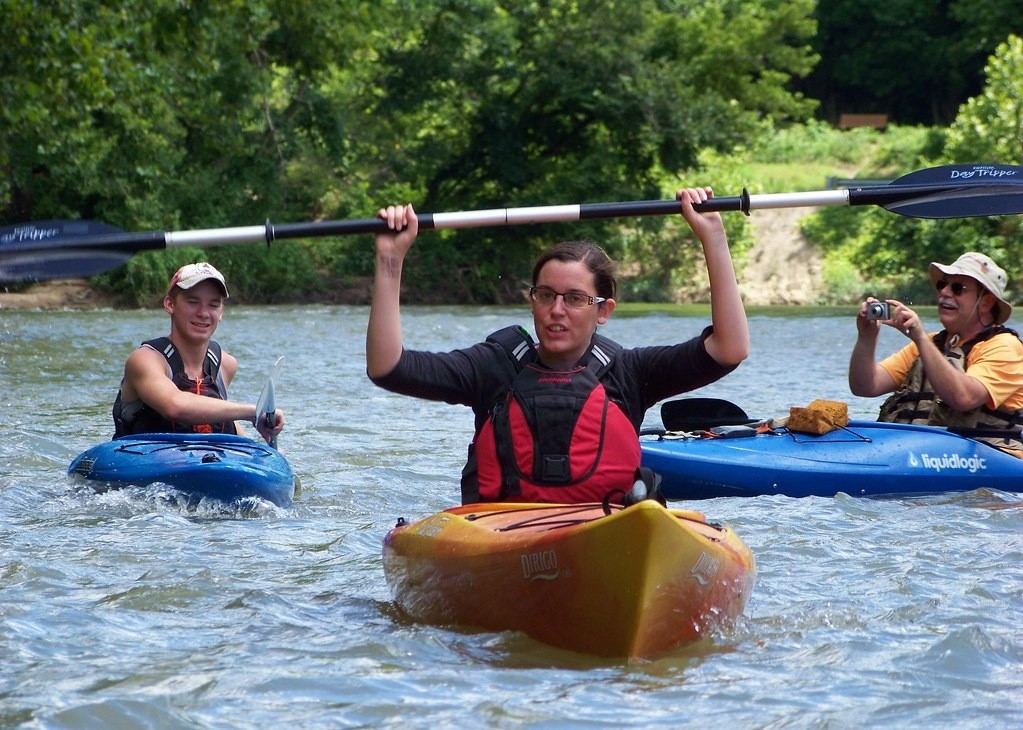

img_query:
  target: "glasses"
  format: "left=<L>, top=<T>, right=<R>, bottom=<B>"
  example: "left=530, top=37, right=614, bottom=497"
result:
left=935, top=280, right=984, bottom=297
left=529, top=286, right=606, bottom=308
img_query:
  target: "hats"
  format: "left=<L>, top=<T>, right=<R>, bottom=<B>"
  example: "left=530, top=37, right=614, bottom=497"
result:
left=168, top=262, right=229, bottom=302
left=929, top=252, right=1012, bottom=326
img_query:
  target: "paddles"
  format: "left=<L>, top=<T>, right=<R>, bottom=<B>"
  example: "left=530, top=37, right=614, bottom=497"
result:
left=1, top=164, right=1023, bottom=285
left=253, top=355, right=280, bottom=456
left=661, top=397, right=1023, bottom=440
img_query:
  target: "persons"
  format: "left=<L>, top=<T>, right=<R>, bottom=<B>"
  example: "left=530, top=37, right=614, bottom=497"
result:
left=365, top=187, right=750, bottom=507
left=112, top=263, right=284, bottom=456
left=848, top=251, right=1023, bottom=458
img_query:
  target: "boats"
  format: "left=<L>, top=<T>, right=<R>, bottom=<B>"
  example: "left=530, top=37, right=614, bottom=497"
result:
left=639, top=418, right=1023, bottom=500
left=381, top=499, right=757, bottom=661
left=64, top=433, right=295, bottom=510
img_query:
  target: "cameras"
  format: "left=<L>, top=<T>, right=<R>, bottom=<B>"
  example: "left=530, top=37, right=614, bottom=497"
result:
left=867, top=303, right=890, bottom=320
